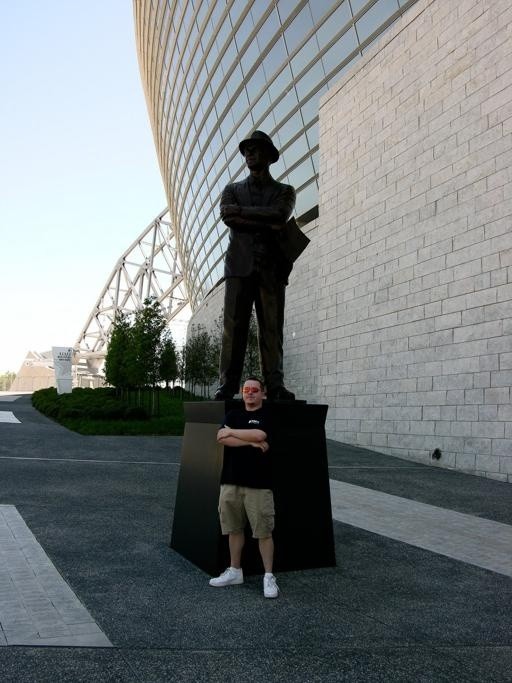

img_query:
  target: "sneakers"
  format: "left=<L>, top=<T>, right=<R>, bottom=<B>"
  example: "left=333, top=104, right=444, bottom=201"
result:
left=263, top=576, right=278, bottom=597
left=209, top=567, right=244, bottom=587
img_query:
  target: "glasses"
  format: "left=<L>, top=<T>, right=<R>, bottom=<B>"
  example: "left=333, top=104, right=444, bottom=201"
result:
left=242, top=387, right=261, bottom=393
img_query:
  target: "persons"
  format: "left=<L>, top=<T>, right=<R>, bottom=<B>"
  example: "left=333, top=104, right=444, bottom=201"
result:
left=208, top=377, right=281, bottom=599
left=213, top=130, right=295, bottom=400
